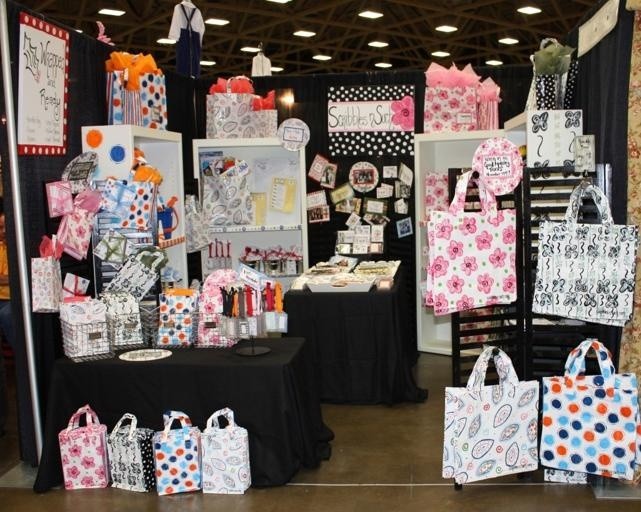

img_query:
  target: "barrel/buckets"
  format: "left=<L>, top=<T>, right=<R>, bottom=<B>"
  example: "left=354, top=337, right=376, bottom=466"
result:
left=158, top=207, right=180, bottom=238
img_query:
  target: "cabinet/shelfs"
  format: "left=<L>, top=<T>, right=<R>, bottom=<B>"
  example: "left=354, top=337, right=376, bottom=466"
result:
left=412, top=130, right=533, bottom=361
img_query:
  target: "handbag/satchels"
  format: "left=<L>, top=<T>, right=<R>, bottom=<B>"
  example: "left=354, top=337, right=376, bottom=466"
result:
left=539, top=337, right=641, bottom=481
left=27, top=163, right=165, bottom=314
left=105, top=51, right=168, bottom=130
left=529, top=38, right=579, bottom=111
left=531, top=180, right=639, bottom=328
left=425, top=169, right=518, bottom=317
left=205, top=76, right=278, bottom=139
left=60, top=268, right=240, bottom=358
left=202, top=155, right=253, bottom=226
left=207, top=238, right=303, bottom=276
left=441, top=346, right=539, bottom=485
left=58, top=404, right=251, bottom=495
left=423, top=81, right=500, bottom=134
left=184, top=194, right=213, bottom=254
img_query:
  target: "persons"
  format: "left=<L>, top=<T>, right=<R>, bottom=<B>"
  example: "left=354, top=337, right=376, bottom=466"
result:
left=322, top=167, right=335, bottom=184
left=311, top=212, right=315, bottom=220
left=363, top=175, right=369, bottom=183
left=316, top=208, right=324, bottom=221
left=402, top=223, right=411, bottom=235
left=358, top=175, right=365, bottom=183
left=367, top=171, right=372, bottom=181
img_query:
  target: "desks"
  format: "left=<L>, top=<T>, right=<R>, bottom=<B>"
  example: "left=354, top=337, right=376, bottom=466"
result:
left=50, top=335, right=309, bottom=486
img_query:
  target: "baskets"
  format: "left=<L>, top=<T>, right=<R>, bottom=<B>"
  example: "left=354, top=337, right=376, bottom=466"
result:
left=58, top=306, right=242, bottom=363
